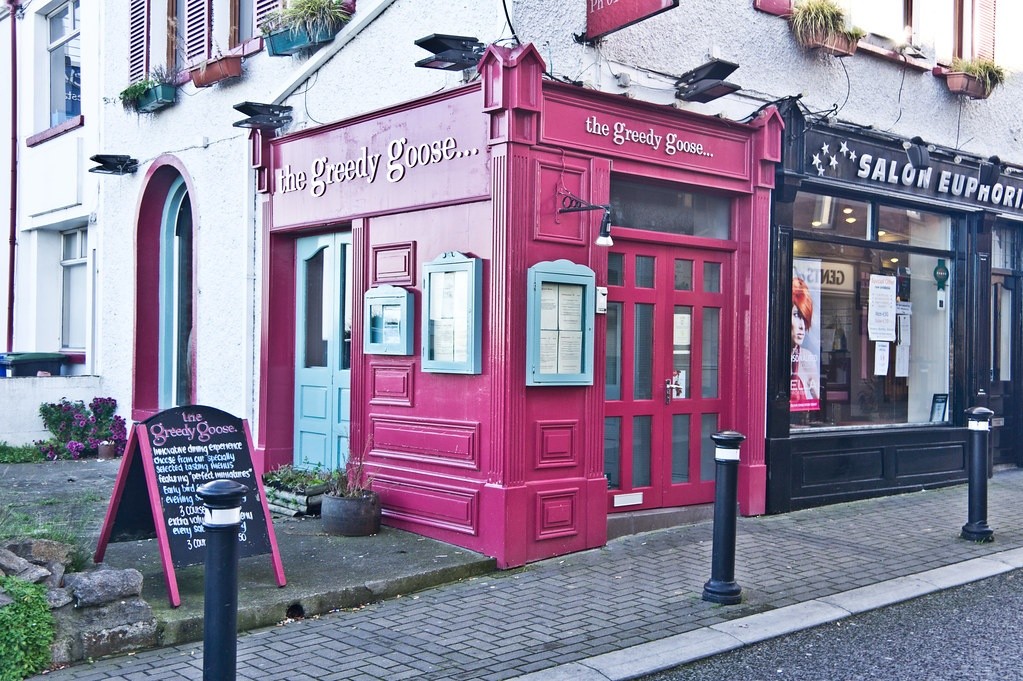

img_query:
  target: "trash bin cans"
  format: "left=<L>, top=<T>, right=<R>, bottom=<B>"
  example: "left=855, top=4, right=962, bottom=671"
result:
left=0, top=353, right=8, bottom=378
left=0, top=351, right=71, bottom=377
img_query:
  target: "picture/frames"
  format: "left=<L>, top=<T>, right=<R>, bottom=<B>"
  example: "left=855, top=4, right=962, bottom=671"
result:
left=929, top=394, right=949, bottom=422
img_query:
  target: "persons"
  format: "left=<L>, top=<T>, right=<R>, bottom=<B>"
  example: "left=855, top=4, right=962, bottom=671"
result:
left=789, top=278, right=819, bottom=400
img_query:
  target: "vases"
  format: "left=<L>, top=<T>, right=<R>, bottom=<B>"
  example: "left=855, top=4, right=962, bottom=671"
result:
left=97, top=444, right=116, bottom=458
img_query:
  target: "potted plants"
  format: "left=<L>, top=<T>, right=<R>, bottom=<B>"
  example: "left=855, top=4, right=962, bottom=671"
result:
left=946, top=56, right=1006, bottom=99
left=317, top=460, right=380, bottom=537
left=188, top=54, right=243, bottom=88
left=789, top=0, right=865, bottom=57
left=259, top=0, right=342, bottom=57
left=120, top=80, right=176, bottom=114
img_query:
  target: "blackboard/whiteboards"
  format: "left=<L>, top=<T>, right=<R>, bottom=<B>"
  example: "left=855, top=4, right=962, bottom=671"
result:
left=100, top=404, right=277, bottom=570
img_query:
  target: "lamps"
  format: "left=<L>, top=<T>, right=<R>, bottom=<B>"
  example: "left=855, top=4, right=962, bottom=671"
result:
left=977, top=156, right=999, bottom=185
left=89, top=153, right=138, bottom=166
left=413, top=33, right=484, bottom=52
left=233, top=114, right=291, bottom=131
left=672, top=58, right=739, bottom=84
left=415, top=50, right=481, bottom=72
left=902, top=136, right=930, bottom=171
left=680, top=81, right=741, bottom=103
left=560, top=194, right=614, bottom=248
left=88, top=163, right=138, bottom=176
left=232, top=101, right=293, bottom=116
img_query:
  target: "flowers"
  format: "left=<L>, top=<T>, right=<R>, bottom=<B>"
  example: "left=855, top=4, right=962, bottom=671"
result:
left=33, top=395, right=124, bottom=459
